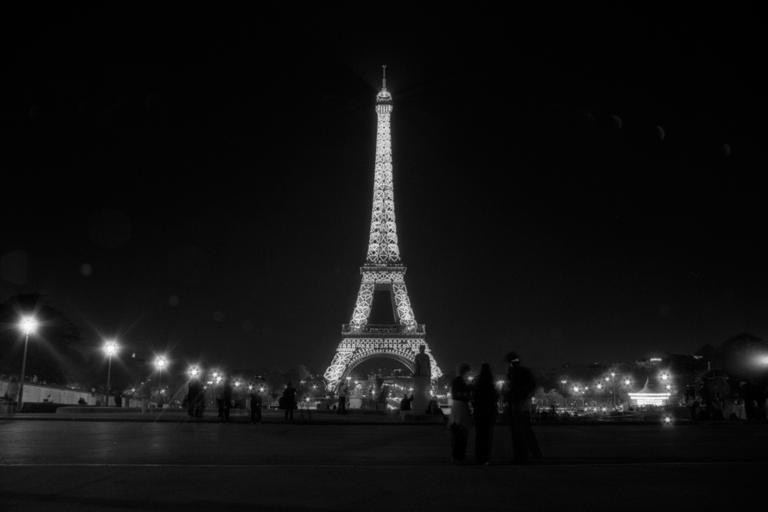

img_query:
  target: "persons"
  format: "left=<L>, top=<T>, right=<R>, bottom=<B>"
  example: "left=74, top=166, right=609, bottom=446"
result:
left=428, top=395, right=448, bottom=419
left=401, top=392, right=414, bottom=410
left=216, top=375, right=234, bottom=425
left=249, top=380, right=263, bottom=425
left=470, top=361, right=501, bottom=461
left=187, top=377, right=203, bottom=416
left=505, top=349, right=545, bottom=463
left=281, top=379, right=297, bottom=423
left=337, top=381, right=349, bottom=414
left=451, top=364, right=472, bottom=455
left=5, top=376, right=19, bottom=414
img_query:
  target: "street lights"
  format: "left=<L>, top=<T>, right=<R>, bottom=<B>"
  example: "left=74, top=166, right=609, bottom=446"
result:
left=16, top=313, right=41, bottom=412
left=103, top=340, right=121, bottom=406
left=154, top=355, right=172, bottom=397
left=659, top=369, right=671, bottom=405
left=187, top=364, right=269, bottom=409
left=560, top=370, right=634, bottom=411
